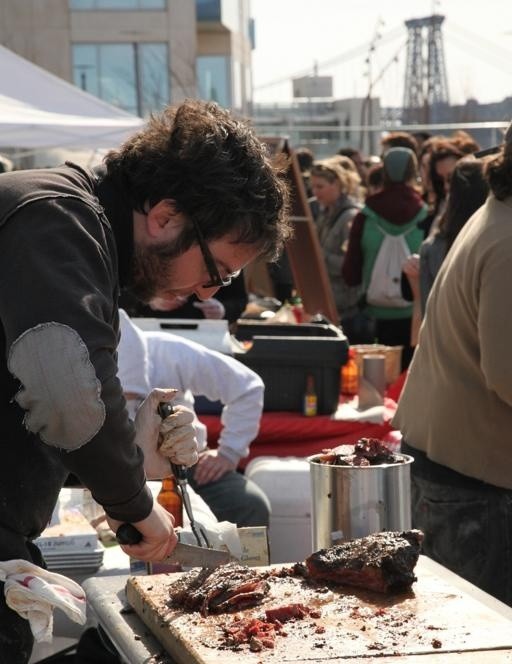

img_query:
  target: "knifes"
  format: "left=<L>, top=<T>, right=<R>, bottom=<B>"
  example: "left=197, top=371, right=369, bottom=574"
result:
left=113, top=523, right=239, bottom=575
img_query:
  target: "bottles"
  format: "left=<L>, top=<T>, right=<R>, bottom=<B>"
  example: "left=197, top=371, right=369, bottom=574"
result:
left=301, top=373, right=318, bottom=416
left=340, top=349, right=360, bottom=397
left=154, top=477, right=183, bottom=530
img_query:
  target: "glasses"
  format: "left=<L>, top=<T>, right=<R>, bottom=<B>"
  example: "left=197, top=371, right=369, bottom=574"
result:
left=180, top=203, right=234, bottom=289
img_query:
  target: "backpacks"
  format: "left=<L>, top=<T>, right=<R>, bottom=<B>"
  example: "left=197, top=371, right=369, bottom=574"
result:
left=355, top=209, right=420, bottom=310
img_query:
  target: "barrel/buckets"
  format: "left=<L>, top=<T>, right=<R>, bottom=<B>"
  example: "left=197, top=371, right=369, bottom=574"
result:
left=305, top=452, right=414, bottom=552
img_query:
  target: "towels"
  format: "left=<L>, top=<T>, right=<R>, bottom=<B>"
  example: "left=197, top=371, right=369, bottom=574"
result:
left=0, top=557, right=89, bottom=644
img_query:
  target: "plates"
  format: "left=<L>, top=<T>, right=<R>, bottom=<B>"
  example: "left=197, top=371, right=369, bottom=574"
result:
left=41, top=540, right=105, bottom=569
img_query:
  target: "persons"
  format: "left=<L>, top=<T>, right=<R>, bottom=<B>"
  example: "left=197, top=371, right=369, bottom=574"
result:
left=272, top=131, right=491, bottom=372
left=0, top=95, right=295, bottom=664
left=99, top=299, right=271, bottom=549
left=390, top=126, right=512, bottom=608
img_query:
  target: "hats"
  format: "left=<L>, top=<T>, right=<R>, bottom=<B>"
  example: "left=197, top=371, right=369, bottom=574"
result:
left=380, top=145, right=418, bottom=184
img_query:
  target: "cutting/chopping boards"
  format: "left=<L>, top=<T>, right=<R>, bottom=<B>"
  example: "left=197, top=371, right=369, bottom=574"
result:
left=121, top=550, right=512, bottom=661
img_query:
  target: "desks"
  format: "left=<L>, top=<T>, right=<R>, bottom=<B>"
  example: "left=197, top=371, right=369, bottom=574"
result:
left=78, top=554, right=512, bottom=662
